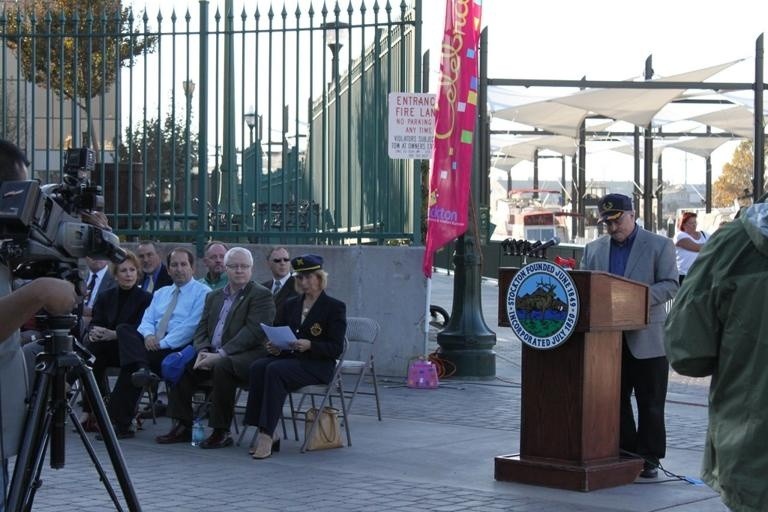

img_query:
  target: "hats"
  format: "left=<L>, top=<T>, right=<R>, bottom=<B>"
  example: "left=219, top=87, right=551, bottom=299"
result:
left=161, top=345, right=195, bottom=383
left=291, top=254, right=323, bottom=276
left=597, top=194, right=633, bottom=223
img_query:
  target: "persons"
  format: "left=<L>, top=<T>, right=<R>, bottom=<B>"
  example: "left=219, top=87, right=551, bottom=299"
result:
left=135, top=242, right=174, bottom=292
left=195, top=241, right=231, bottom=290
left=663, top=191, right=768, bottom=512
left=242, top=254, right=347, bottom=458
left=91, top=247, right=216, bottom=439
left=581, top=192, right=680, bottom=477
left=71, top=253, right=155, bottom=433
left=156, top=247, right=278, bottom=450
left=78, top=254, right=116, bottom=325
left=671, top=211, right=713, bottom=287
left=0, top=139, right=83, bottom=482
left=259, top=248, right=297, bottom=300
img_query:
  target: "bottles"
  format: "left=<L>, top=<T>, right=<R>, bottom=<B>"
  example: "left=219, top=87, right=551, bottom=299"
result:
left=191, top=418, right=204, bottom=447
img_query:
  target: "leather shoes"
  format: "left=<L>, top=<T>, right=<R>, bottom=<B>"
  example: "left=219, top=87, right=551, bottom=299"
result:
left=639, top=461, right=658, bottom=477
left=73, top=411, right=280, bottom=459
left=131, top=369, right=160, bottom=386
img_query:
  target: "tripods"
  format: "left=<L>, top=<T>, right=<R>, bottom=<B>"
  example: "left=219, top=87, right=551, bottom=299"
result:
left=5, top=333, right=142, bottom=511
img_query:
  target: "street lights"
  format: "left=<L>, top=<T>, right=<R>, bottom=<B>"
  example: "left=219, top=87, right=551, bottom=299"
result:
left=241, top=109, right=261, bottom=156
left=317, top=22, right=354, bottom=232
left=179, top=79, right=200, bottom=216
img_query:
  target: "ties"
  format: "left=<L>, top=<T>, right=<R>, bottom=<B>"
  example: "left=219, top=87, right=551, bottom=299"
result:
left=147, top=275, right=154, bottom=293
left=83, top=274, right=97, bottom=306
left=156, top=288, right=179, bottom=340
left=274, top=281, right=280, bottom=294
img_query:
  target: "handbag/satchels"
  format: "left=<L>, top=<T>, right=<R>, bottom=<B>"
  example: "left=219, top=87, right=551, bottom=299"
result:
left=407, top=361, right=438, bottom=389
left=306, top=406, right=345, bottom=450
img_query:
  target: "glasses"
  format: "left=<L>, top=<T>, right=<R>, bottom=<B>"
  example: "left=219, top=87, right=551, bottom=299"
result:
left=273, top=258, right=290, bottom=263
left=226, top=265, right=250, bottom=271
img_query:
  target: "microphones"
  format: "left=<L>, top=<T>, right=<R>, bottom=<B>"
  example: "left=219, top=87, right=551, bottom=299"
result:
left=530, top=236, right=561, bottom=253
